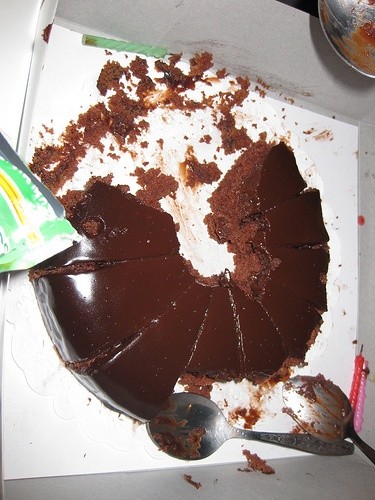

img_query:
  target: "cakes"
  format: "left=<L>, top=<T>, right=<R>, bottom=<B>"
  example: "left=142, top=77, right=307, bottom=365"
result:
left=30, top=50, right=332, bottom=424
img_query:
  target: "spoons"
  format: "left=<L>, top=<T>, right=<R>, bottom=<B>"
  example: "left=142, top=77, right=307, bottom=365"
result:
left=282, top=376, right=374, bottom=463
left=148, top=393, right=355, bottom=460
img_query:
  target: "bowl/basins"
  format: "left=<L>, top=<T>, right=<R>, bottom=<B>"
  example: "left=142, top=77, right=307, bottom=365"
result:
left=318, top=0, right=375, bottom=78
